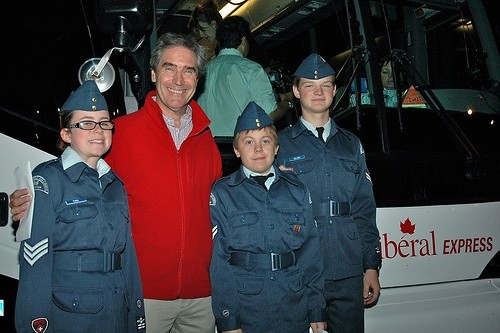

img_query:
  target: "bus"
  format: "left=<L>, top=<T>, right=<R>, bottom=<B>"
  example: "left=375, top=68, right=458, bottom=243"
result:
left=0, top=0, right=500, bottom=333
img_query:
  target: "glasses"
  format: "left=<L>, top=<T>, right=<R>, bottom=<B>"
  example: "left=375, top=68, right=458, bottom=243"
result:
left=65, top=121, right=115, bottom=130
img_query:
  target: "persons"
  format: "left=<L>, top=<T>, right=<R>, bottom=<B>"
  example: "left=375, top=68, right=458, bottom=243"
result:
left=349, top=55, right=398, bottom=108
left=197, top=15, right=290, bottom=177
left=189, top=0, right=269, bottom=68
left=10, top=32, right=295, bottom=333
left=275, top=54, right=382, bottom=333
left=15, top=81, right=146, bottom=333
left=209, top=101, right=327, bottom=333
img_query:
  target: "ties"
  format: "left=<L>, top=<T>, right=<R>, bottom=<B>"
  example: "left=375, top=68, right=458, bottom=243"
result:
left=249, top=173, right=274, bottom=191
left=316, top=127, right=324, bottom=143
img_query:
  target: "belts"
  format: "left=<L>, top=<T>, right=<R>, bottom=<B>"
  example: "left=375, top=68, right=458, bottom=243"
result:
left=53, top=252, right=121, bottom=273
left=230, top=249, right=300, bottom=271
left=313, top=200, right=352, bottom=217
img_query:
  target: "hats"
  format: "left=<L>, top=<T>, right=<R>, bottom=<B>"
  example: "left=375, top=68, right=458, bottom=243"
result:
left=61, top=80, right=108, bottom=110
left=235, top=101, right=274, bottom=132
left=294, top=54, right=336, bottom=80
